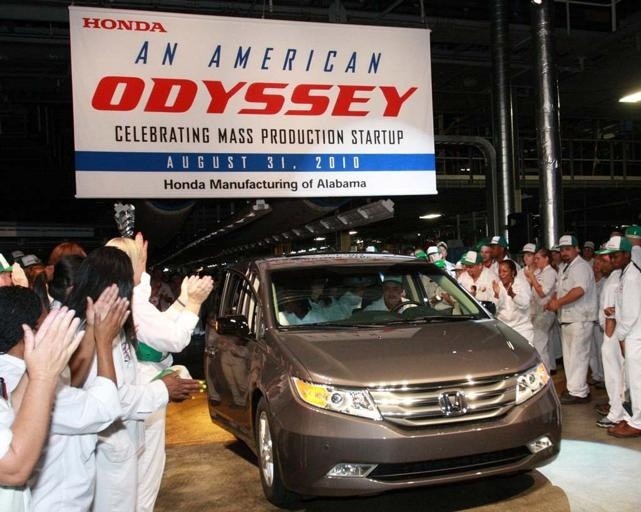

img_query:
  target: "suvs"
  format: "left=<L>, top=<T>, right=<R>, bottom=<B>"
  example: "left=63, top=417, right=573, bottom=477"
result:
left=202, top=250, right=566, bottom=511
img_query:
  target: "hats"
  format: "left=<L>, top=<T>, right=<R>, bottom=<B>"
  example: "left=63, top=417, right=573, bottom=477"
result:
left=426, top=246, right=441, bottom=256
left=550, top=244, right=560, bottom=252
left=0, top=249, right=14, bottom=273
left=594, top=236, right=631, bottom=255
left=624, top=226, right=641, bottom=239
left=436, top=240, right=448, bottom=249
left=382, top=273, right=403, bottom=284
left=451, top=260, right=463, bottom=271
left=460, top=253, right=465, bottom=264
left=464, top=250, right=482, bottom=265
left=583, top=241, right=594, bottom=249
left=555, top=235, right=578, bottom=248
left=415, top=252, right=428, bottom=261
left=488, top=235, right=507, bottom=248
left=18, top=254, right=40, bottom=268
left=519, top=243, right=539, bottom=253
left=365, top=246, right=378, bottom=253
left=12, top=250, right=23, bottom=259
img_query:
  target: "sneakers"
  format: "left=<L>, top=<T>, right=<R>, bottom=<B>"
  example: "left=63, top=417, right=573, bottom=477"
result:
left=562, top=389, right=591, bottom=397
left=595, top=403, right=632, bottom=416
left=596, top=418, right=615, bottom=428
left=561, top=394, right=589, bottom=404
left=595, top=382, right=605, bottom=389
left=587, top=378, right=600, bottom=384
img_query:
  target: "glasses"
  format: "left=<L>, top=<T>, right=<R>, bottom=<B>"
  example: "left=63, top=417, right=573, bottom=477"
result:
left=584, top=248, right=593, bottom=252
left=311, top=278, right=329, bottom=286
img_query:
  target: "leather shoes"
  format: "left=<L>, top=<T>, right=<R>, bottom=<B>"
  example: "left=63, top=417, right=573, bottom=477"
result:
left=613, top=425, right=640, bottom=438
left=607, top=420, right=626, bottom=435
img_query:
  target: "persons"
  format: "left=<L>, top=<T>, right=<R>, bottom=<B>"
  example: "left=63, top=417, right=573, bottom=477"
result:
left=593, top=235, right=640, bottom=439
left=0, top=301, right=88, bottom=512
left=544, top=233, right=597, bottom=405
left=365, top=225, right=641, bottom=428
left=492, top=260, right=533, bottom=343
left=1, top=233, right=223, bottom=512
left=274, top=270, right=420, bottom=329
